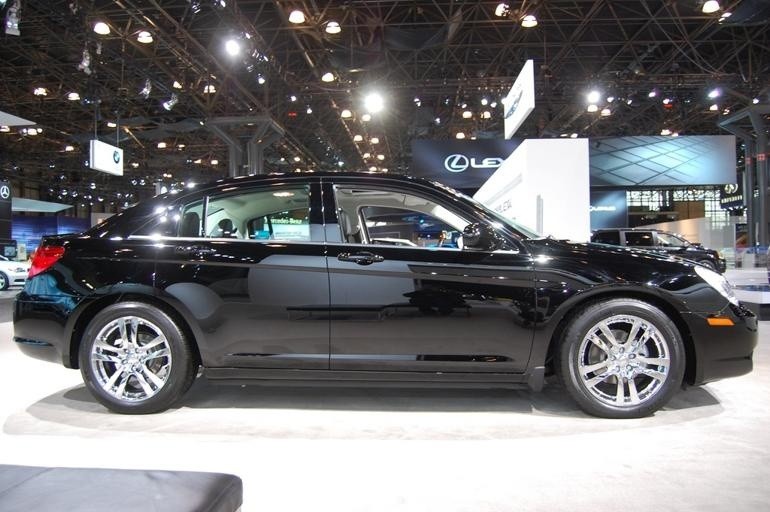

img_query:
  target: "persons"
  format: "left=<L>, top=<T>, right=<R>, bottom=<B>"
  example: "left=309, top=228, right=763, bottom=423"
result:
left=437, top=232, right=448, bottom=247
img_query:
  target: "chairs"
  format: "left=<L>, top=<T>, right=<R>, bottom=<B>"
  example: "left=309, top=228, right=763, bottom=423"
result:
left=340, top=210, right=351, bottom=244
left=209, top=220, right=234, bottom=239
left=179, top=212, right=200, bottom=237
